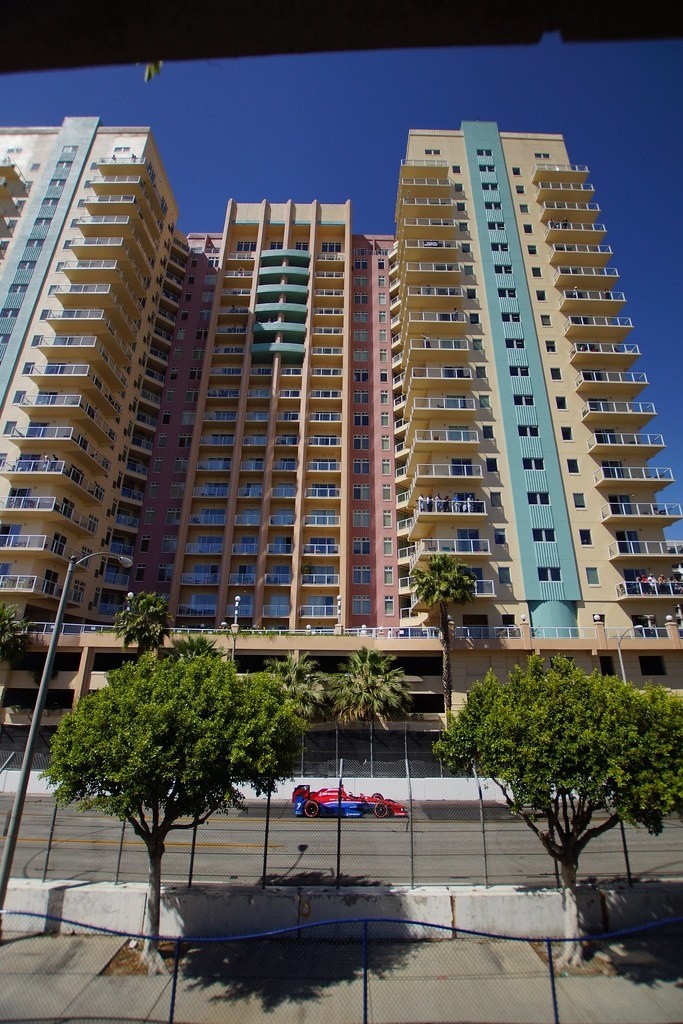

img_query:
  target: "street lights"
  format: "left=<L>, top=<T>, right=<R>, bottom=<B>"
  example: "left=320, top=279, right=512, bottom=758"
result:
left=618, top=624, right=642, bottom=688
left=0, top=552, right=134, bottom=906
left=337, top=595, right=342, bottom=624
left=230, top=623, right=239, bottom=660
left=233, top=595, right=241, bottom=624
left=126, top=591, right=133, bottom=612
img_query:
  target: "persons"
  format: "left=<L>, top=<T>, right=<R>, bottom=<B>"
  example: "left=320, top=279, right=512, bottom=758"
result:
left=420, top=333, right=431, bottom=347
left=425, top=284, right=432, bottom=295
left=603, top=288, right=612, bottom=299
left=414, top=491, right=484, bottom=514
left=452, top=307, right=460, bottom=321
left=10, top=453, right=61, bottom=471
left=548, top=216, right=570, bottom=229
left=631, top=572, right=683, bottom=596
left=572, top=286, right=580, bottom=298
left=236, top=266, right=245, bottom=276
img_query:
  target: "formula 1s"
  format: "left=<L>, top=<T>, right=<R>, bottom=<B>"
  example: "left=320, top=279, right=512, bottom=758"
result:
left=292, top=785, right=408, bottom=819
